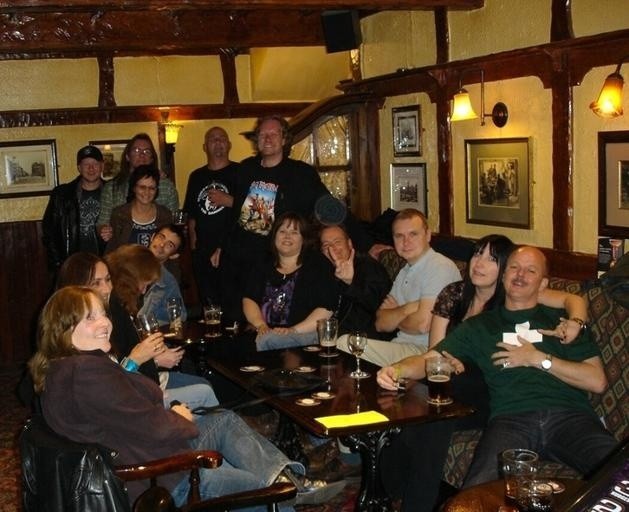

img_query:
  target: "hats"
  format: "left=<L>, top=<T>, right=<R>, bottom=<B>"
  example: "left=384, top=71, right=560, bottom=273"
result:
left=77, top=146, right=103, bottom=166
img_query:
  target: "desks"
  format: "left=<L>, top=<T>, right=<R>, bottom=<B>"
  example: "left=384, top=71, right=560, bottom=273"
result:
left=159, top=320, right=252, bottom=380
left=207, top=344, right=480, bottom=512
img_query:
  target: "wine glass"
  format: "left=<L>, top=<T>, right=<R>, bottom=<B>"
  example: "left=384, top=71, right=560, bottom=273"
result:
left=137, top=311, right=168, bottom=352
left=348, top=328, right=370, bottom=379
left=208, top=179, right=223, bottom=192
left=350, top=379, right=368, bottom=413
left=168, top=296, right=184, bottom=338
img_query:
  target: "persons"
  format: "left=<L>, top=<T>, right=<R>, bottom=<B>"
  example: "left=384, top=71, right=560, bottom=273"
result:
left=59, top=252, right=311, bottom=469
left=310, top=209, right=462, bottom=474
left=109, top=223, right=210, bottom=390
left=29, top=284, right=348, bottom=512
left=353, top=235, right=587, bottom=512
left=209, top=113, right=394, bottom=294
left=41, top=146, right=106, bottom=276
left=95, top=133, right=178, bottom=242
left=182, top=127, right=246, bottom=300
left=240, top=210, right=341, bottom=350
left=477, top=158, right=517, bottom=207
left=315, top=223, right=393, bottom=341
left=106, top=164, right=172, bottom=250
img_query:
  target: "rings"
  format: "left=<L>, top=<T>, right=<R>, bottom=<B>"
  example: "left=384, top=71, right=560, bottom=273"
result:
left=154, top=346, right=159, bottom=350
left=505, top=359, right=509, bottom=364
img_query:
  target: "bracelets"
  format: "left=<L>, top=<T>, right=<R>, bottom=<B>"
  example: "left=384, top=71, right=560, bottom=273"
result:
left=573, top=318, right=584, bottom=326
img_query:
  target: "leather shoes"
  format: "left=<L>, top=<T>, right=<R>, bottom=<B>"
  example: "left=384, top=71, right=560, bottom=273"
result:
left=275, top=471, right=347, bottom=509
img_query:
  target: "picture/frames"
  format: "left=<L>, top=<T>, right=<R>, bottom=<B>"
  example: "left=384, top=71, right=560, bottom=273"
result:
left=392, top=105, right=425, bottom=157
left=389, top=163, right=428, bottom=219
left=0, top=139, right=59, bottom=199
left=465, top=138, right=536, bottom=229
left=89, top=140, right=129, bottom=181
left=598, top=131, right=629, bottom=239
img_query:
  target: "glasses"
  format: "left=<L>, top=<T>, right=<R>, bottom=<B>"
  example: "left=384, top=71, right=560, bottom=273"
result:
left=131, top=147, right=153, bottom=157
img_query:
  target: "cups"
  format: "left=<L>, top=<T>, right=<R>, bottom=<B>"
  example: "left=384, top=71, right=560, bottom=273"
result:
left=173, top=209, right=186, bottom=225
left=318, top=357, right=337, bottom=390
left=427, top=403, right=449, bottom=415
left=425, top=357, right=452, bottom=404
left=528, top=484, right=555, bottom=512
left=502, top=448, right=539, bottom=498
left=316, top=318, right=339, bottom=355
left=204, top=309, right=220, bottom=324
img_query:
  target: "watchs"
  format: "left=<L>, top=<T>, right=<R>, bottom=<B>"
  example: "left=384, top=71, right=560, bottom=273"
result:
left=541, top=353, right=552, bottom=372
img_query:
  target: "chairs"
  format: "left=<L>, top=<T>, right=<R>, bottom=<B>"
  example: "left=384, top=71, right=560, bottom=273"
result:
left=112, top=450, right=298, bottom=512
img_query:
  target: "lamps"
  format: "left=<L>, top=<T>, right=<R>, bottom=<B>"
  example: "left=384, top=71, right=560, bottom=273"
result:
left=162, top=120, right=184, bottom=165
left=449, top=68, right=508, bottom=127
left=589, top=63, right=624, bottom=120
